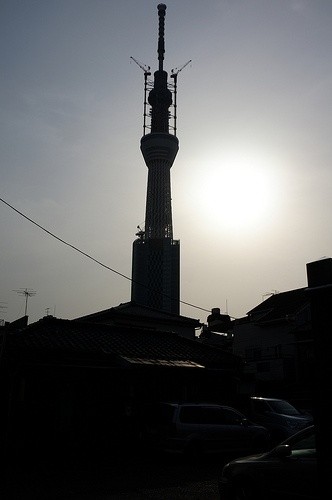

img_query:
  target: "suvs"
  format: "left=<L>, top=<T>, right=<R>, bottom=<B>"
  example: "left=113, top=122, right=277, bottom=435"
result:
left=150, top=403, right=271, bottom=459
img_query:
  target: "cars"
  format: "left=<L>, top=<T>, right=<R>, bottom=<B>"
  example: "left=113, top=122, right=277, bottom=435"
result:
left=219, top=425, right=317, bottom=499
left=248, top=396, right=314, bottom=438
